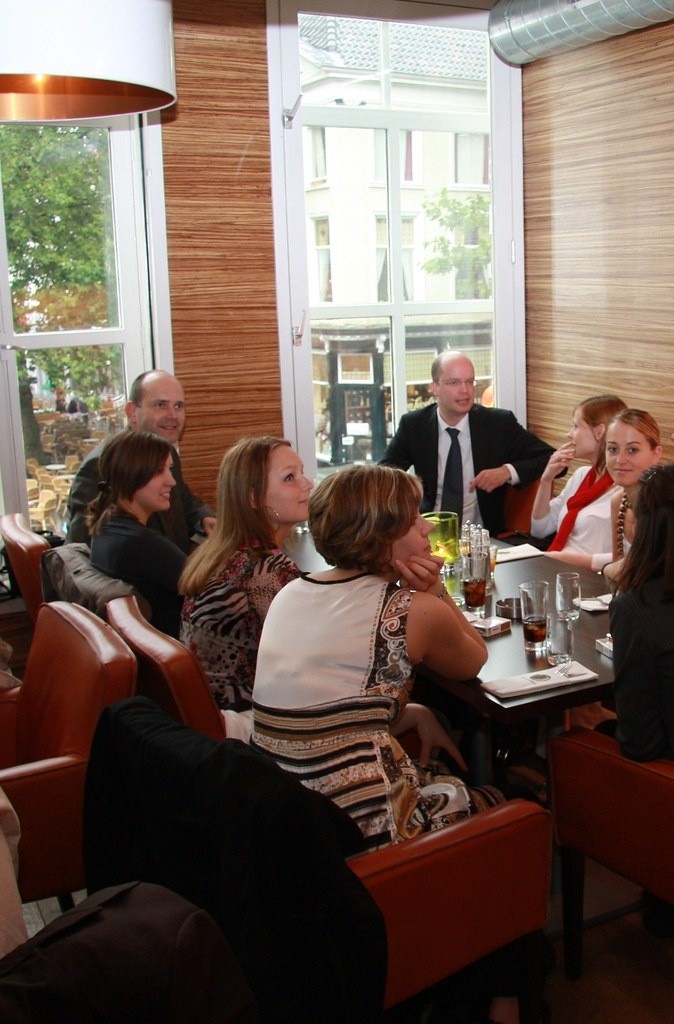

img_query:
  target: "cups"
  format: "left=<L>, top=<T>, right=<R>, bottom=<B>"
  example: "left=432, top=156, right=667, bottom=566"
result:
left=443, top=564, right=464, bottom=607
left=420, top=511, right=461, bottom=572
left=489, top=544, right=498, bottom=579
left=555, top=572, right=580, bottom=622
left=519, top=581, right=551, bottom=653
left=546, top=612, right=574, bottom=666
left=460, top=552, right=488, bottom=614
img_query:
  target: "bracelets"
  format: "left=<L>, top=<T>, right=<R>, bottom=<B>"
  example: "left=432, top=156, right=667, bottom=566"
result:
left=601, top=562, right=613, bottom=576
left=437, top=583, right=447, bottom=598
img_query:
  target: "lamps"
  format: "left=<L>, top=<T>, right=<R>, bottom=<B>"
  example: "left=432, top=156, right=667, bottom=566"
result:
left=0, top=0, right=179, bottom=128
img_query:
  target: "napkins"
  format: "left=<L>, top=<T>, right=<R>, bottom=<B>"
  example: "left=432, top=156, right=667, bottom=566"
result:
left=479, top=660, right=598, bottom=699
left=462, top=611, right=478, bottom=622
left=494, top=543, right=545, bottom=563
left=571, top=593, right=613, bottom=612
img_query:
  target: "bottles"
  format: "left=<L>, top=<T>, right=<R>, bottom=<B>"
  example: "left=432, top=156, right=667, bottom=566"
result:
left=460, top=521, right=490, bottom=555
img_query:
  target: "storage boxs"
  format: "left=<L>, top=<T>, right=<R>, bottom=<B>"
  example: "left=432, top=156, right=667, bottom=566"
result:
left=471, top=616, right=512, bottom=637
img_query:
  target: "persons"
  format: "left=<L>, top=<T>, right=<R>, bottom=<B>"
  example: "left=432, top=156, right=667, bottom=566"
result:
left=571, top=463, right=674, bottom=939
left=177, top=436, right=315, bottom=712
left=249, top=465, right=553, bottom=1024
left=531, top=392, right=661, bottom=585
left=378, top=351, right=569, bottom=538
left=66, top=371, right=216, bottom=640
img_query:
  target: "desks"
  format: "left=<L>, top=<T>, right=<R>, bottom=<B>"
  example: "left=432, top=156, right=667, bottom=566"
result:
left=281, top=518, right=618, bottom=774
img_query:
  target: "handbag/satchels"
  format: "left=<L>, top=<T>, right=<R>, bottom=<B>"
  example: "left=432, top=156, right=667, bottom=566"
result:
left=0, top=530, right=65, bottom=600
left=487, top=521, right=546, bottom=553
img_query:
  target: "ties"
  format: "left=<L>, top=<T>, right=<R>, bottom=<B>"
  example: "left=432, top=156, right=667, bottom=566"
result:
left=438, top=427, right=463, bottom=540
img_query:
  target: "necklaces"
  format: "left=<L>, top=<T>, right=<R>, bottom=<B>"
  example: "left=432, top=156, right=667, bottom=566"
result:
left=616, top=492, right=633, bottom=560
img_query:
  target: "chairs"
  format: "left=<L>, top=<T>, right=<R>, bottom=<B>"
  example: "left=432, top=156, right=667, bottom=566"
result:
left=481, top=478, right=554, bottom=547
left=0, top=512, right=52, bottom=630
left=540, top=721, right=674, bottom=951
left=0, top=599, right=140, bottom=913
left=104, top=593, right=560, bottom=1024
left=24, top=395, right=129, bottom=536
left=40, top=543, right=134, bottom=621
